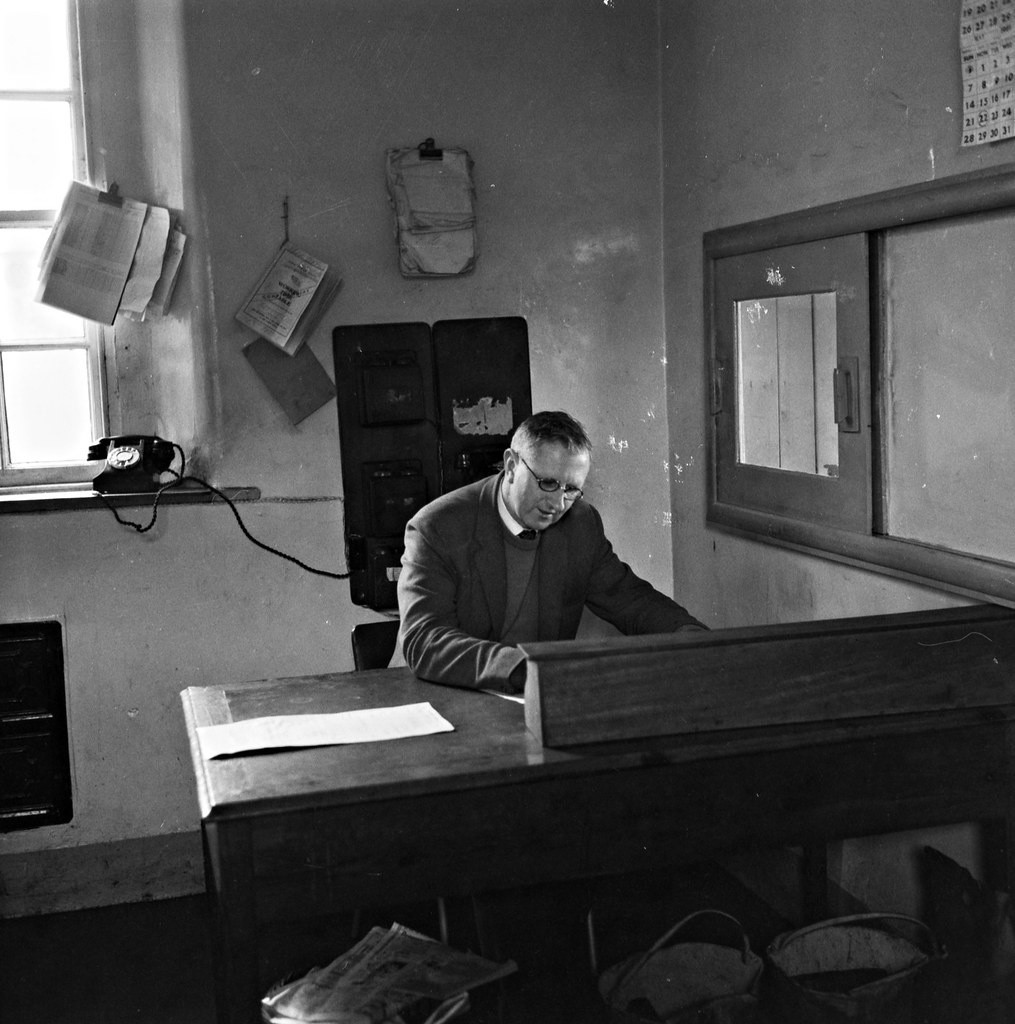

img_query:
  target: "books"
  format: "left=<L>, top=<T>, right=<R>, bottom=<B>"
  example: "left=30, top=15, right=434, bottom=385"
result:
left=235, top=241, right=344, bottom=358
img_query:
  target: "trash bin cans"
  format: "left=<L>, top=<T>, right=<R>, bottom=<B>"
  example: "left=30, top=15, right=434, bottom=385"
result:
left=264, top=965, right=472, bottom=1024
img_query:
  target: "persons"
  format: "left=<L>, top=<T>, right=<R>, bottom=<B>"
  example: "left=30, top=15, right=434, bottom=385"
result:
left=390, top=411, right=715, bottom=695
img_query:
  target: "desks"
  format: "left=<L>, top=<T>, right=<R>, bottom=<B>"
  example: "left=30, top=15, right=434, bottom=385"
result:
left=179, top=663, right=1015, bottom=1024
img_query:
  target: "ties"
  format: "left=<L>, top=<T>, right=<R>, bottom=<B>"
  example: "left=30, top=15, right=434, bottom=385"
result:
left=518, top=529, right=537, bottom=541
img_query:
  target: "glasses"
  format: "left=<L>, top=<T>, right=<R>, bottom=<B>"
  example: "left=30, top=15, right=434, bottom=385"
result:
left=517, top=454, right=584, bottom=500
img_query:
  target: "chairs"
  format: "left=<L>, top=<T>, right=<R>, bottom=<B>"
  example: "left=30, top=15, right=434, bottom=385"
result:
left=351, top=619, right=617, bottom=993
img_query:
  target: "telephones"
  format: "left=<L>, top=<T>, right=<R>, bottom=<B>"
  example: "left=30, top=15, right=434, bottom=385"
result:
left=87, top=435, right=175, bottom=493
